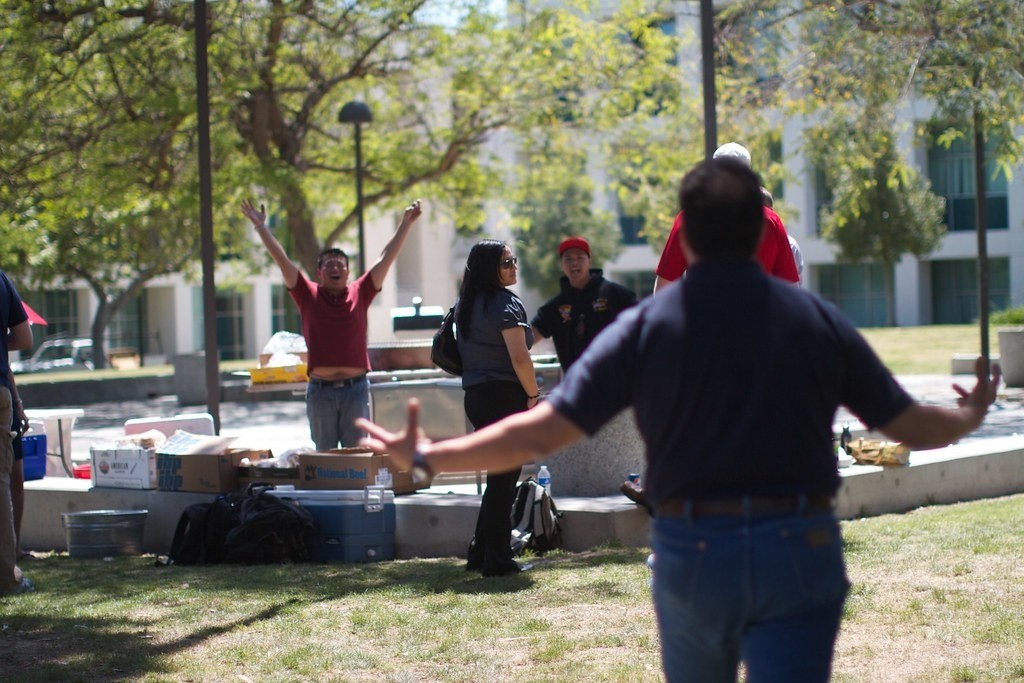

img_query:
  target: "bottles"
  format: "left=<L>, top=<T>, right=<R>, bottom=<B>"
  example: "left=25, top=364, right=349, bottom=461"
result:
left=537, top=466, right=551, bottom=497
left=833, top=432, right=838, bottom=457
left=841, top=425, right=852, bottom=454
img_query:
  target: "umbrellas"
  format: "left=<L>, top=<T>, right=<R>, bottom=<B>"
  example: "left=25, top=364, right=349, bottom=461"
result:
left=21, top=300, right=47, bottom=328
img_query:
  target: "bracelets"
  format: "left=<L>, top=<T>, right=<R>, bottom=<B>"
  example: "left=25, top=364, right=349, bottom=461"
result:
left=527, top=389, right=540, bottom=399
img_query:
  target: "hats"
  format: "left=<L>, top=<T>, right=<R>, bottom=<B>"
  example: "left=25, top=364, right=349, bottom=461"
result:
left=713, top=142, right=750, bottom=171
left=559, top=236, right=590, bottom=257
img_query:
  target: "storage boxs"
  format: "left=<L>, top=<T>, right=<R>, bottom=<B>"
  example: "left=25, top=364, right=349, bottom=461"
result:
left=250, top=363, right=309, bottom=386
left=21, top=419, right=48, bottom=480
left=155, top=431, right=278, bottom=494
left=91, top=440, right=157, bottom=489
left=126, top=413, right=217, bottom=442
left=298, top=454, right=416, bottom=496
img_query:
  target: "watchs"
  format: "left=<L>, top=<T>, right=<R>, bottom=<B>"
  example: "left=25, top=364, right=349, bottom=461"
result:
left=410, top=440, right=434, bottom=489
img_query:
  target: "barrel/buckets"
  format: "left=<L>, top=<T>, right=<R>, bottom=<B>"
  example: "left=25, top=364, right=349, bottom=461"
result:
left=21, top=420, right=46, bottom=481
left=73, top=464, right=91, bottom=478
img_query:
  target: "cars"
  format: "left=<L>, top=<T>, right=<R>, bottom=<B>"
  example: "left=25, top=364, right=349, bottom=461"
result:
left=107, top=347, right=141, bottom=370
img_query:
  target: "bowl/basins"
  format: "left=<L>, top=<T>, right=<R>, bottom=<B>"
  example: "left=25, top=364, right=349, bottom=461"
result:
left=62, top=509, right=148, bottom=557
left=838, top=456, right=856, bottom=468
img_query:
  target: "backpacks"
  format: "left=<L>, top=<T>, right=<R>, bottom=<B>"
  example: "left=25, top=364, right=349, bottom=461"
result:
left=510, top=475, right=563, bottom=555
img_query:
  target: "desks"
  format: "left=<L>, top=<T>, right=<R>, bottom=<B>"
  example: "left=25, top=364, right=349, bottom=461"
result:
left=24, top=409, right=85, bottom=477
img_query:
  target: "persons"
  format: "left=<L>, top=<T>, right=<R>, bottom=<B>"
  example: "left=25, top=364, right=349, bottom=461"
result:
left=529, top=236, right=639, bottom=379
left=649, top=141, right=805, bottom=296
left=239, top=198, right=423, bottom=453
left=355, top=155, right=1003, bottom=683
left=0, top=261, right=34, bottom=589
left=449, top=238, right=541, bottom=578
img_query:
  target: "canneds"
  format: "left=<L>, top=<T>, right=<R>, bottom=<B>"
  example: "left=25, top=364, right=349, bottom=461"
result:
left=629, top=473, right=641, bottom=487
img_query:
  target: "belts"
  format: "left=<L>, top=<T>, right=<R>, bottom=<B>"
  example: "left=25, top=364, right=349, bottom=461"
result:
left=309, top=373, right=365, bottom=388
left=656, top=492, right=832, bottom=517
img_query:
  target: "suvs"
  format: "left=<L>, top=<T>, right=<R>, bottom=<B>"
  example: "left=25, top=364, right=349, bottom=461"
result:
left=10, top=339, right=96, bottom=372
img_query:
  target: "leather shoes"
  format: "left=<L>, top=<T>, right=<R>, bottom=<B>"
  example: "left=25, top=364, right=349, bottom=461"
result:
left=621, top=481, right=655, bottom=517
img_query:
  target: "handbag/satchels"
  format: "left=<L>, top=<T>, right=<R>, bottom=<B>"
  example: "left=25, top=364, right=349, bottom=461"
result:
left=431, top=303, right=463, bottom=376
left=168, top=481, right=316, bottom=564
left=847, top=437, right=910, bottom=466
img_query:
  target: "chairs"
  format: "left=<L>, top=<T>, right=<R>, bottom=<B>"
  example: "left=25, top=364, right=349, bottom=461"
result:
left=369, top=379, right=482, bottom=496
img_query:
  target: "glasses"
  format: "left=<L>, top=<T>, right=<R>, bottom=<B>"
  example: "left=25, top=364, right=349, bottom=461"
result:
left=500, top=257, right=517, bottom=269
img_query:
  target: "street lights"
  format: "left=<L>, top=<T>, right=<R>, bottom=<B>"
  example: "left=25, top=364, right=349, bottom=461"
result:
left=338, top=100, right=377, bottom=277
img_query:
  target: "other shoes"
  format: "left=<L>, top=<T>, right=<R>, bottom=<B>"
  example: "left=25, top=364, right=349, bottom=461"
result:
left=466, top=562, right=485, bottom=572
left=0, top=578, right=35, bottom=596
left=482, top=560, right=533, bottom=577
